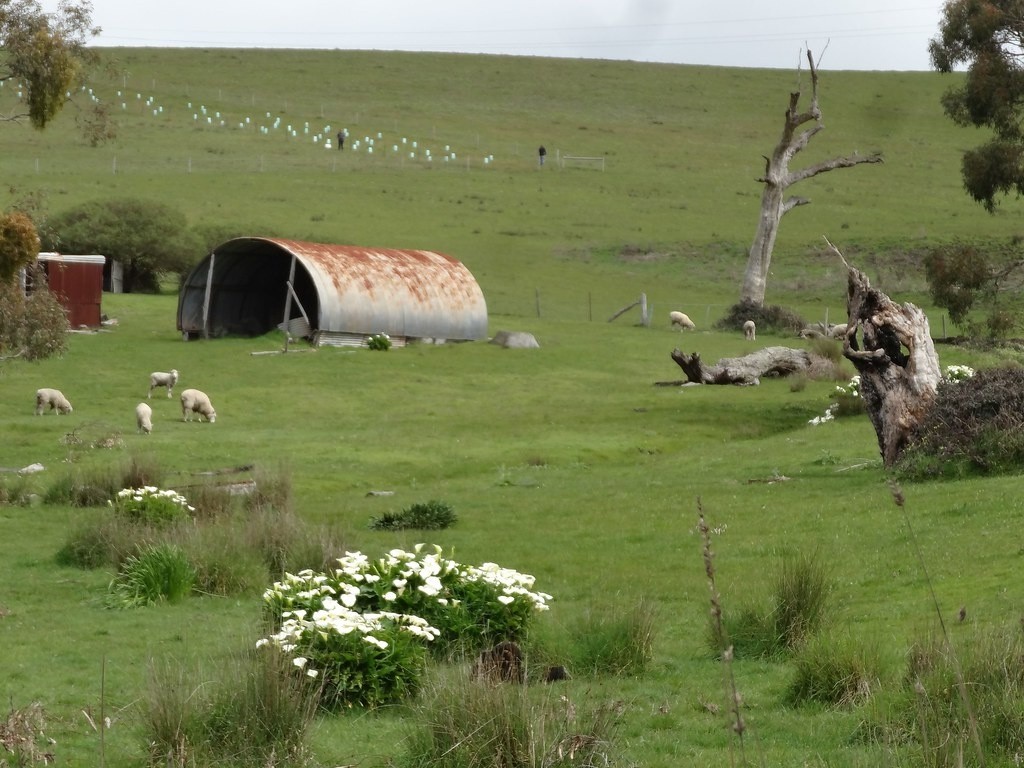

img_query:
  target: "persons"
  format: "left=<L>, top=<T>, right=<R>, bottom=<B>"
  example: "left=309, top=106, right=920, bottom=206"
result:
left=539, top=145, right=546, bottom=165
left=337, top=128, right=345, bottom=150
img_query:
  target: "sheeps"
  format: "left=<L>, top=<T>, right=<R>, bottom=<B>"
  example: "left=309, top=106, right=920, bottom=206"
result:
left=180, top=388, right=217, bottom=423
left=743, top=320, right=756, bottom=341
left=136, top=402, right=154, bottom=434
left=147, top=368, right=179, bottom=399
left=799, top=323, right=848, bottom=341
left=34, top=388, right=73, bottom=418
left=669, top=311, right=695, bottom=332
